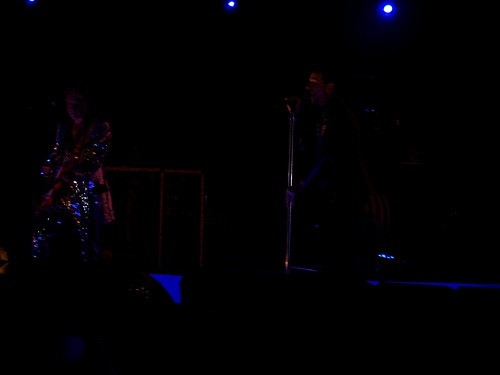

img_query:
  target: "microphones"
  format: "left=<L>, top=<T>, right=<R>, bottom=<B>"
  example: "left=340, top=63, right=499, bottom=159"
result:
left=269, top=91, right=312, bottom=115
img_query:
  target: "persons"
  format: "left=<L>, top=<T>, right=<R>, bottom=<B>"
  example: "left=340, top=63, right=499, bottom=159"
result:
left=30, top=76, right=114, bottom=270
left=286, top=71, right=373, bottom=272
